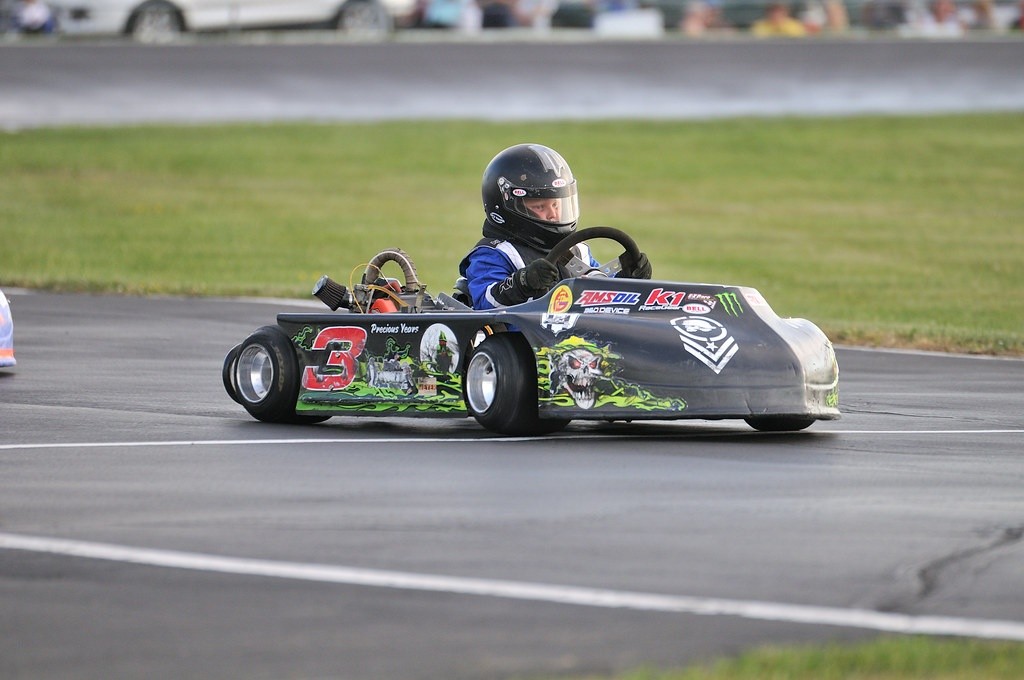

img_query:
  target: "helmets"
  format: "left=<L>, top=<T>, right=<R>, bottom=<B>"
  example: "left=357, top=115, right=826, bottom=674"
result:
left=482, top=143, right=580, bottom=253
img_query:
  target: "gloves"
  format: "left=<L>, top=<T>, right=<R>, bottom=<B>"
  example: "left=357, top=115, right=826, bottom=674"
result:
left=620, top=249, right=652, bottom=279
left=516, top=258, right=560, bottom=299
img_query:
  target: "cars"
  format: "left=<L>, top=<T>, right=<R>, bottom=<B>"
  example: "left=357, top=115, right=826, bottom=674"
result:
left=51, top=1, right=417, bottom=37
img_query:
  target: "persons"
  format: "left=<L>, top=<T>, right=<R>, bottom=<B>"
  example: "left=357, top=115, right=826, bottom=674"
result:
left=460, top=143, right=652, bottom=310
left=422, top=0, right=996, bottom=39
